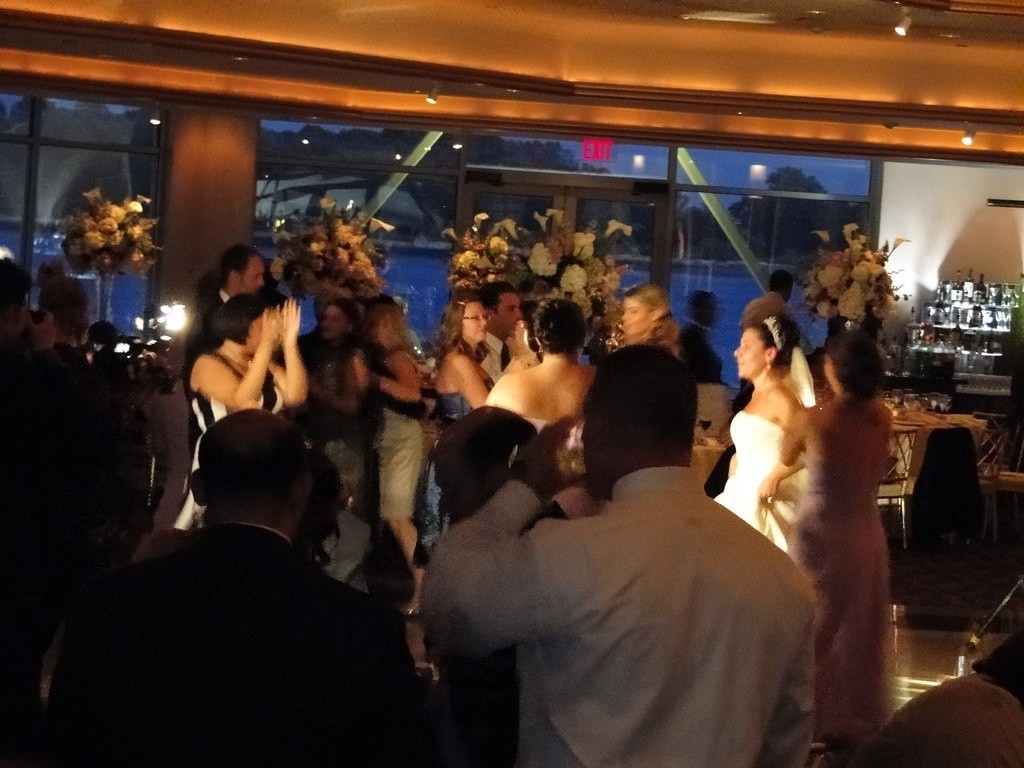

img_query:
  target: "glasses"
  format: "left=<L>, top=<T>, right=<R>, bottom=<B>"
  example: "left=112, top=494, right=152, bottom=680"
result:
left=462, top=314, right=491, bottom=322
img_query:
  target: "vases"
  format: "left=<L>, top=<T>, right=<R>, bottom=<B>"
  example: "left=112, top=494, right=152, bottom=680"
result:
left=95, top=268, right=113, bottom=323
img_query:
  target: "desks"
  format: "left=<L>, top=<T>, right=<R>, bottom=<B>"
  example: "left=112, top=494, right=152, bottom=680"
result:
left=882, top=413, right=988, bottom=540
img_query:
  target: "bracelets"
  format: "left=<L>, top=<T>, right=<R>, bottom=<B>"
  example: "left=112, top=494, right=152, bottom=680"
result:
left=381, top=377, right=392, bottom=393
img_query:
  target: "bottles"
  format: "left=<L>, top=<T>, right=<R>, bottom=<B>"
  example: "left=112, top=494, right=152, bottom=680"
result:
left=890, top=268, right=1024, bottom=375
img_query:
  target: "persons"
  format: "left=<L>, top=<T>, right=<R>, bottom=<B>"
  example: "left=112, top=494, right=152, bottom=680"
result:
left=846, top=628, right=1024, bottom=768
left=0, top=257, right=176, bottom=768
left=181, top=243, right=883, bottom=619
left=781, top=328, right=891, bottom=744
left=62, top=409, right=439, bottom=768
left=420, top=341, right=814, bottom=768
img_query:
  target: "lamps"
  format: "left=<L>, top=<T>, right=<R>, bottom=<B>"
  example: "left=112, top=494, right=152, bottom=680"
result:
left=426, top=87, right=440, bottom=105
left=894, top=6, right=912, bottom=37
left=961, top=128, right=976, bottom=146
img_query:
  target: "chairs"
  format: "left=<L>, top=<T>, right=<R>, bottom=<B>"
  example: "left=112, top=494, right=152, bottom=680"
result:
left=874, top=412, right=1024, bottom=553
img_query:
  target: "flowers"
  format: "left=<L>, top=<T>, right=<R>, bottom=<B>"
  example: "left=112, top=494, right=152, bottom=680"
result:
left=801, top=223, right=914, bottom=323
left=267, top=197, right=395, bottom=299
left=441, top=208, right=633, bottom=336
left=56, top=188, right=164, bottom=277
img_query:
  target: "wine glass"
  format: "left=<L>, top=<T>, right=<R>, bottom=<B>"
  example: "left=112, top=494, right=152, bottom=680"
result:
left=875, top=388, right=952, bottom=420
left=700, top=415, right=713, bottom=447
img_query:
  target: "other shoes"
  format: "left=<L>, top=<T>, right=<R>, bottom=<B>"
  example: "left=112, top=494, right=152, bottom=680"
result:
left=403, top=602, right=422, bottom=615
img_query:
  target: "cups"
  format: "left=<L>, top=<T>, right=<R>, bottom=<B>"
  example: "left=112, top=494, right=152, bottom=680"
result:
left=556, top=419, right=585, bottom=474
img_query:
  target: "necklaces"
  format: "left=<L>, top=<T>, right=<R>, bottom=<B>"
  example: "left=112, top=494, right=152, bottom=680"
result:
left=222, top=348, right=251, bottom=369
